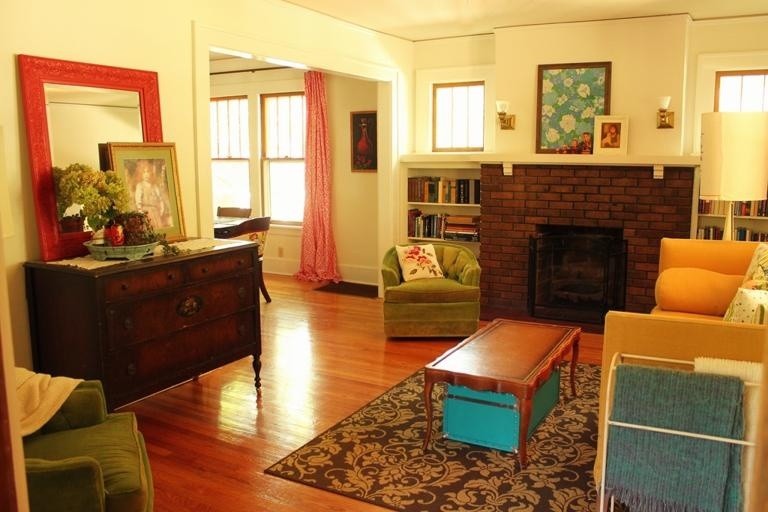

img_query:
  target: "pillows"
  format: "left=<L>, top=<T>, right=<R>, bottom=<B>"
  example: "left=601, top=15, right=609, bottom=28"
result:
left=395, top=243, right=445, bottom=282
left=720, top=242, right=767, bottom=325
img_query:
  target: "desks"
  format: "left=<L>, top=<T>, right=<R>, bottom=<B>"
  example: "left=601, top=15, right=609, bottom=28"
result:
left=214, top=216, right=254, bottom=237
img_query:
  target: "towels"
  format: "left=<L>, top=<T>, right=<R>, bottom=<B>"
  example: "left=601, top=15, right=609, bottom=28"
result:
left=43, top=237, right=240, bottom=272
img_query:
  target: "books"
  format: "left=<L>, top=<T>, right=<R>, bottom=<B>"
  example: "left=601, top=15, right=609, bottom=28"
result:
left=697, top=199, right=768, bottom=241
left=408, top=176, right=480, bottom=243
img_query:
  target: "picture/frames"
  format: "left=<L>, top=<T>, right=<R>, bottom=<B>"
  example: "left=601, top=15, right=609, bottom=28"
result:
left=536, top=60, right=611, bottom=160
left=349, top=110, right=378, bottom=172
left=101, top=140, right=187, bottom=244
left=591, top=113, right=631, bottom=162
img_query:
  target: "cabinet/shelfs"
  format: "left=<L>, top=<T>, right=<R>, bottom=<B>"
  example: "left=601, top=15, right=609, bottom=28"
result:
left=689, top=184, right=768, bottom=241
left=23, top=239, right=261, bottom=410
left=406, top=166, right=481, bottom=243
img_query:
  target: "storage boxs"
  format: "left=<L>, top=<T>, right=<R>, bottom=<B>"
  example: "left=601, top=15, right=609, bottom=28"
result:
left=445, top=371, right=559, bottom=453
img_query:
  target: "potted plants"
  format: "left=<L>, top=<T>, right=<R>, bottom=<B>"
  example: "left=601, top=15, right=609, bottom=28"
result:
left=52, top=163, right=86, bottom=231
left=55, top=164, right=184, bottom=260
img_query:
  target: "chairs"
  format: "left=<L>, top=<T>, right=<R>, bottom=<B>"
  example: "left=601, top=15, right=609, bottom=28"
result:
left=217, top=205, right=253, bottom=218
left=379, top=243, right=484, bottom=340
left=225, top=217, right=273, bottom=303
left=15, top=368, right=158, bottom=511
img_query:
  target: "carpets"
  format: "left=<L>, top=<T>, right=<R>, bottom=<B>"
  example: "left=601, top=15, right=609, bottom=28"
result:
left=262, top=355, right=603, bottom=512
left=314, top=280, right=380, bottom=300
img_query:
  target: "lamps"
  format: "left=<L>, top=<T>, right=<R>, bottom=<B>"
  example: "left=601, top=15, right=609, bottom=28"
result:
left=494, top=98, right=517, bottom=131
left=699, top=111, right=768, bottom=241
left=655, top=95, right=676, bottom=131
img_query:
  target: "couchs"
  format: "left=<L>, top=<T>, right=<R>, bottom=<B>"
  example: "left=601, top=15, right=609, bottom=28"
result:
left=593, top=235, right=768, bottom=511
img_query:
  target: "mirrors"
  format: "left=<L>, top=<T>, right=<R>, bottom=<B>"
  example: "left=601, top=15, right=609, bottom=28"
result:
left=15, top=54, right=166, bottom=262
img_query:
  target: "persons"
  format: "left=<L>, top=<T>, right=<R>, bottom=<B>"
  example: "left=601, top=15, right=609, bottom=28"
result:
left=602, top=125, right=620, bottom=148
left=556, top=130, right=593, bottom=154
left=356, top=117, right=373, bottom=155
left=131, top=159, right=168, bottom=233
